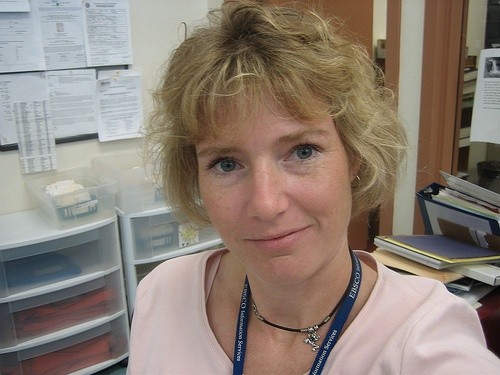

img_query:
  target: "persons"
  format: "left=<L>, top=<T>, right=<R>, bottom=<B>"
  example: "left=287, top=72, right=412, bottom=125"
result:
left=125, top=0, right=500, bottom=375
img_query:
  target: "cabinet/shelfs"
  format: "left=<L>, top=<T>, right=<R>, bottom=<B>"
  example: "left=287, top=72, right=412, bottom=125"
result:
left=0, top=183, right=224, bottom=375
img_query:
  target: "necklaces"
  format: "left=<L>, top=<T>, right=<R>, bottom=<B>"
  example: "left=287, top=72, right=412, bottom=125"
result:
left=252, top=245, right=356, bottom=351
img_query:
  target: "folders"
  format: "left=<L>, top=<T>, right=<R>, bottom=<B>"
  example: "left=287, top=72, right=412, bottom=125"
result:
left=416, top=182, right=500, bottom=251
